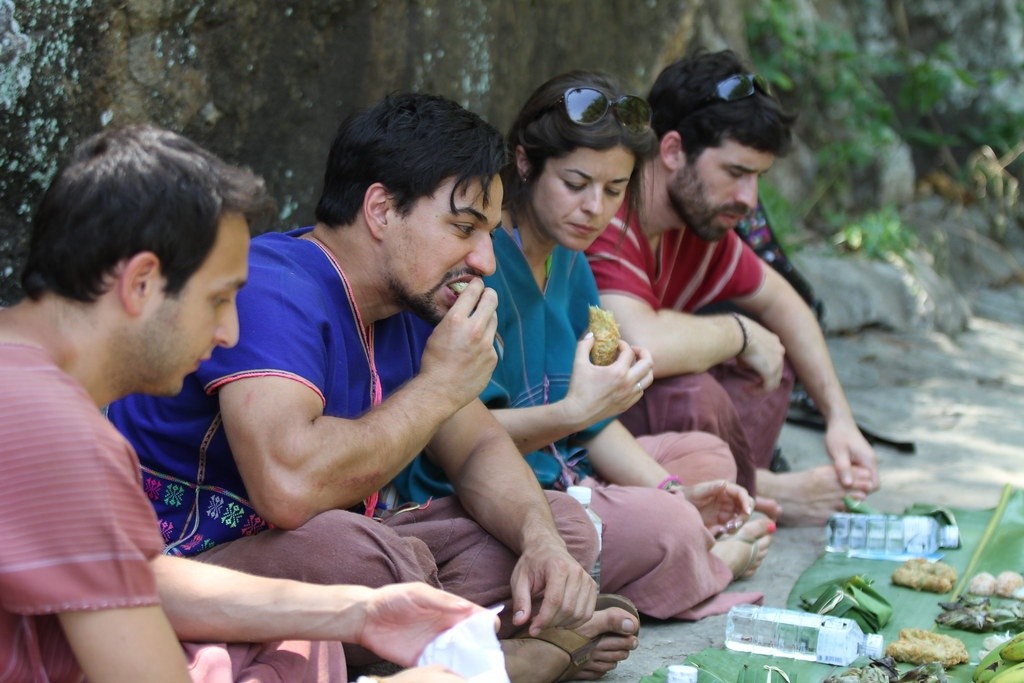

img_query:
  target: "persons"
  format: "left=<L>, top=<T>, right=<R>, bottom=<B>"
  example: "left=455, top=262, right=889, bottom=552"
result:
left=579, top=48, right=879, bottom=531
left=0, top=120, right=504, bottom=683
left=98, top=93, right=639, bottom=683
left=391, top=71, right=779, bottom=626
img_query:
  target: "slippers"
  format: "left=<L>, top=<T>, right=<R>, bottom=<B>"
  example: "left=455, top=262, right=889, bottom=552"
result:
left=715, top=512, right=772, bottom=581
left=515, top=593, right=640, bottom=683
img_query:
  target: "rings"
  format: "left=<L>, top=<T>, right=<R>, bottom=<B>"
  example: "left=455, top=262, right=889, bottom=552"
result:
left=636, top=382, right=643, bottom=392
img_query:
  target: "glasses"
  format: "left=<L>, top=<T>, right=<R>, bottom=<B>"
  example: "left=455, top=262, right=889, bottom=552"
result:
left=524, top=86, right=653, bottom=135
left=672, top=73, right=776, bottom=129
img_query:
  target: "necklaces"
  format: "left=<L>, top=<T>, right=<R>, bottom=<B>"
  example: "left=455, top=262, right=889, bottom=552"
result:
left=508, top=202, right=553, bottom=292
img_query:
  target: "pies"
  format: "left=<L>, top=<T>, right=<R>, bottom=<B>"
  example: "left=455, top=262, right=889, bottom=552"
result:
left=588, top=306, right=622, bottom=364
left=892, top=559, right=957, bottom=594
left=887, top=630, right=968, bottom=669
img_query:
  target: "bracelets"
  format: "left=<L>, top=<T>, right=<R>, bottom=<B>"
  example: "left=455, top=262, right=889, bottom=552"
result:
left=656, top=476, right=682, bottom=492
left=730, top=312, right=753, bottom=357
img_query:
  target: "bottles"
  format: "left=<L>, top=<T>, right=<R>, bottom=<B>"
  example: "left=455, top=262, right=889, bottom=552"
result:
left=667, top=665, right=698, bottom=683
left=824, top=512, right=959, bottom=559
left=566, top=486, right=602, bottom=594
left=724, top=602, right=884, bottom=666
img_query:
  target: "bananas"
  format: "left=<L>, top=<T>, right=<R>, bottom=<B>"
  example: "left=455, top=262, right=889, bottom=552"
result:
left=973, top=631, right=1024, bottom=683
